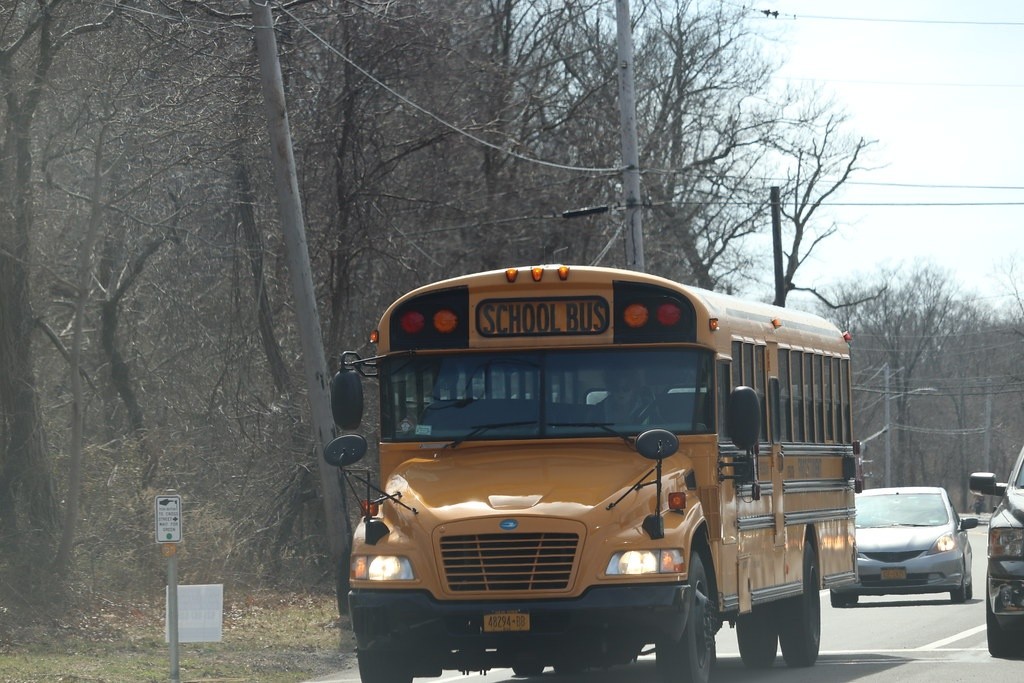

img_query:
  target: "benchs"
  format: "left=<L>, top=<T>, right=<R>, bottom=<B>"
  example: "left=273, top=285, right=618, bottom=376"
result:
left=581, top=390, right=709, bottom=427
left=419, top=399, right=598, bottom=430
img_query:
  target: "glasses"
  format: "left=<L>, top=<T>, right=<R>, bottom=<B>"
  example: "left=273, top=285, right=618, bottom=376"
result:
left=611, top=384, right=631, bottom=393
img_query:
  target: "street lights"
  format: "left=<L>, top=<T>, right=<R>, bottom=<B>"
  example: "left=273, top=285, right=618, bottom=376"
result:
left=882, top=361, right=938, bottom=485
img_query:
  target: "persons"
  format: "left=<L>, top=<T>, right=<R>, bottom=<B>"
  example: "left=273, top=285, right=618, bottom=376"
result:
left=585, top=361, right=676, bottom=426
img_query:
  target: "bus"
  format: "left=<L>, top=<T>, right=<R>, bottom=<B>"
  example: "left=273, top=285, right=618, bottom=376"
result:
left=321, top=262, right=864, bottom=683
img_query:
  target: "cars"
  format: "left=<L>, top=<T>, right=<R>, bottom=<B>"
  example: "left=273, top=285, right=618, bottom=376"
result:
left=830, top=486, right=979, bottom=609
left=968, top=443, right=1024, bottom=660
